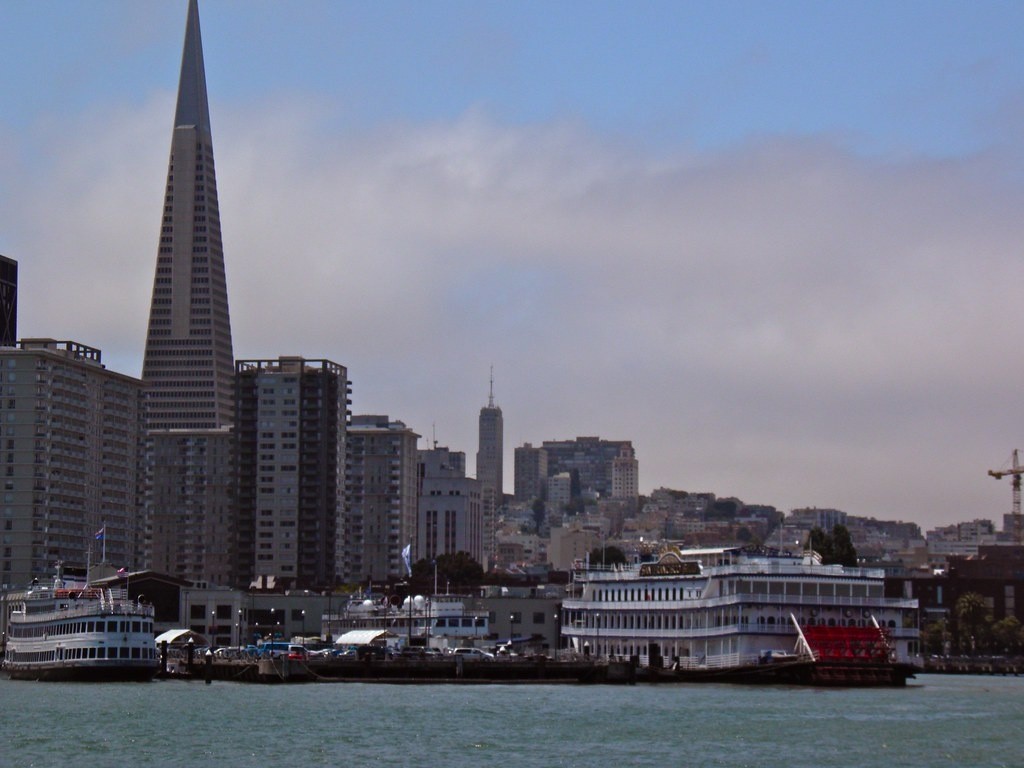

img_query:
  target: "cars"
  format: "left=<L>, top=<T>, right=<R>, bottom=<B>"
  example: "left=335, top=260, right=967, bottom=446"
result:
left=319, top=649, right=368, bottom=661
left=241, top=645, right=259, bottom=658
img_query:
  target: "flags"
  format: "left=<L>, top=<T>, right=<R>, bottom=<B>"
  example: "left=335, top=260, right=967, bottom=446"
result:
left=367, top=584, right=371, bottom=596
left=402, top=544, right=413, bottom=578
left=117, top=567, right=126, bottom=576
left=95, top=528, right=104, bottom=540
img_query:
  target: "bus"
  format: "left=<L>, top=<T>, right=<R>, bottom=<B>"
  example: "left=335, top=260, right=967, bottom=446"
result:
left=258, top=642, right=297, bottom=658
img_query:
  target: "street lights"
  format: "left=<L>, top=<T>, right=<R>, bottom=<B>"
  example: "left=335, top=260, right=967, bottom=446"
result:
left=238, top=608, right=241, bottom=658
left=596, top=612, right=600, bottom=659
left=554, top=614, right=559, bottom=661
left=211, top=611, right=215, bottom=656
left=510, top=615, right=514, bottom=647
left=302, top=609, right=305, bottom=654
left=270, top=607, right=274, bottom=657
left=475, top=615, right=478, bottom=636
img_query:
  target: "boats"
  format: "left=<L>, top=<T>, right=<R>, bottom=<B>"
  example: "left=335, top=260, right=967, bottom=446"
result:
left=1, top=560, right=159, bottom=680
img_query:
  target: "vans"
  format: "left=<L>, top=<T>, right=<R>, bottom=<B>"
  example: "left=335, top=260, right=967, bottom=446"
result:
left=453, top=648, right=494, bottom=662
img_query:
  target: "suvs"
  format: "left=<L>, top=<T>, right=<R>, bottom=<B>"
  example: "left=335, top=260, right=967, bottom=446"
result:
left=358, top=645, right=401, bottom=661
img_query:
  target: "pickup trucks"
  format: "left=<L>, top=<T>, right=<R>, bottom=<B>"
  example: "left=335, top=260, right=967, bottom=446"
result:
left=269, top=645, right=318, bottom=658
left=390, top=646, right=443, bottom=662
